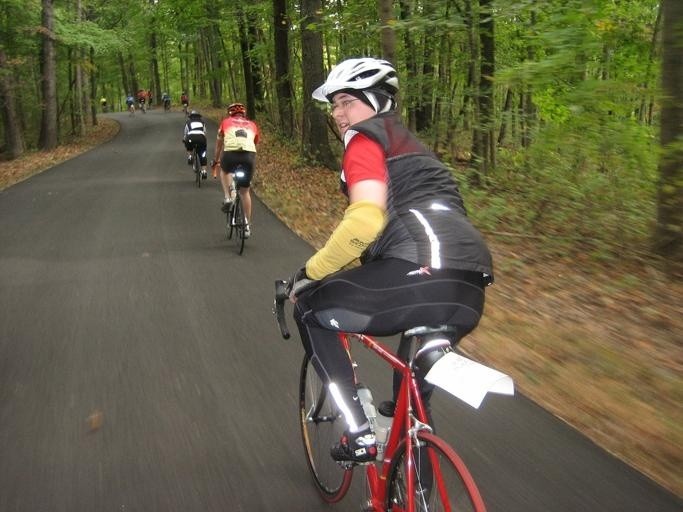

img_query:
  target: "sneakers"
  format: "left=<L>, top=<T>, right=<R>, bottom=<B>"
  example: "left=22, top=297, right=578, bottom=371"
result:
left=245, top=229, right=253, bottom=239
left=331, top=430, right=378, bottom=463
left=188, top=154, right=193, bottom=164
left=221, top=198, right=234, bottom=213
left=202, top=170, right=207, bottom=179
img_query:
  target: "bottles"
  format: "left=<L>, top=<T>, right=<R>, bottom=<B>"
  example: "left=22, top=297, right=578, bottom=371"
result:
left=355, top=381, right=396, bottom=464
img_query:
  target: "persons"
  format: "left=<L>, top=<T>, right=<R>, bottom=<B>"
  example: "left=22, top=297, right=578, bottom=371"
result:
left=125, top=89, right=189, bottom=114
left=214, top=103, right=260, bottom=237
left=182, top=111, right=207, bottom=180
left=99, top=96, right=106, bottom=109
left=281, top=58, right=495, bottom=511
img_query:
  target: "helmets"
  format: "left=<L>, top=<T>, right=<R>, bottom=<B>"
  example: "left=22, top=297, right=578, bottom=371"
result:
left=312, top=58, right=399, bottom=103
left=191, top=110, right=201, bottom=118
left=227, top=103, right=246, bottom=116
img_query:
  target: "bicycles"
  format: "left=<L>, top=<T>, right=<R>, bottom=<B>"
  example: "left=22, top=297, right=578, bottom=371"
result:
left=271, top=275, right=514, bottom=512
left=209, top=158, right=248, bottom=256
left=128, top=97, right=190, bottom=117
left=181, top=137, right=204, bottom=188
left=101, top=104, right=109, bottom=112
